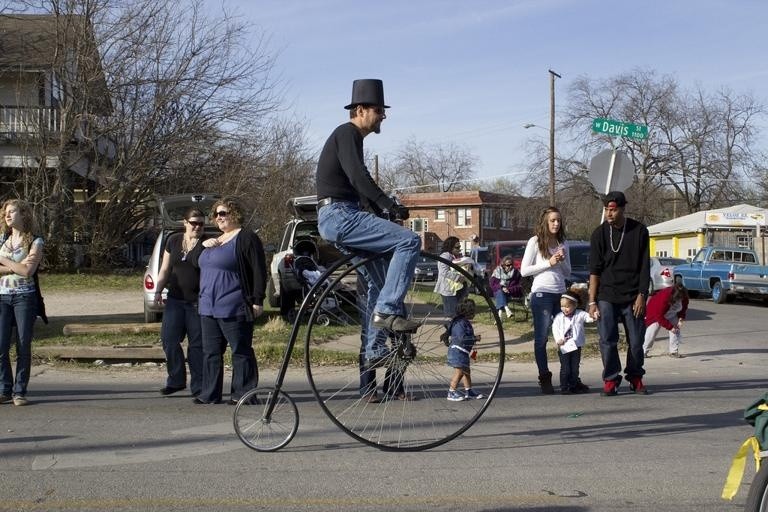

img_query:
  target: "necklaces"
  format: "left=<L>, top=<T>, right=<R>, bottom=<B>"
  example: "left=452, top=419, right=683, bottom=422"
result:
left=181, top=234, right=202, bottom=262
left=610, top=218, right=627, bottom=252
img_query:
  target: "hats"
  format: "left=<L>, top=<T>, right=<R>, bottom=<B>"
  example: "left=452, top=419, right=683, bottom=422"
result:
left=603, top=191, right=628, bottom=207
left=344, top=79, right=391, bottom=109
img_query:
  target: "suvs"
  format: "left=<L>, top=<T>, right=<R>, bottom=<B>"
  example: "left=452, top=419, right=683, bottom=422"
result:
left=266, top=194, right=360, bottom=315
left=142, top=192, right=261, bottom=327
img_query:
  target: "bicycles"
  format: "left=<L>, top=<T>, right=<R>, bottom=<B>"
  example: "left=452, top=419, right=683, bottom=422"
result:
left=232, top=206, right=507, bottom=452
left=744, top=450, right=768, bottom=511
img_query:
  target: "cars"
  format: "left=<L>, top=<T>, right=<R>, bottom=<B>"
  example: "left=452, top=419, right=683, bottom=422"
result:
left=463, top=240, right=593, bottom=299
left=649, top=253, right=690, bottom=300
left=412, top=250, right=442, bottom=283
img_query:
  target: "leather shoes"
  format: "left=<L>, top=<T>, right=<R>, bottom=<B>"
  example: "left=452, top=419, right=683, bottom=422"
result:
left=359, top=350, right=395, bottom=372
left=369, top=313, right=422, bottom=331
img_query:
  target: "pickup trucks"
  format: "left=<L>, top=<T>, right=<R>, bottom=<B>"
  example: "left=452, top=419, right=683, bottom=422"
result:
left=673, top=246, right=767, bottom=304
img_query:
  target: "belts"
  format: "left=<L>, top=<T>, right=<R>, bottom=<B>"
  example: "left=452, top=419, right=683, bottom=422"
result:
left=317, top=197, right=341, bottom=212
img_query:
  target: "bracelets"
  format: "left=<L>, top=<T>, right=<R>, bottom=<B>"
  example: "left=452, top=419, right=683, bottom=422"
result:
left=155, top=291, right=161, bottom=294
left=588, top=302, right=596, bottom=306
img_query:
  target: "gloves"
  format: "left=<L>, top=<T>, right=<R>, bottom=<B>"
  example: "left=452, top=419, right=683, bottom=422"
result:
left=389, top=203, right=410, bottom=220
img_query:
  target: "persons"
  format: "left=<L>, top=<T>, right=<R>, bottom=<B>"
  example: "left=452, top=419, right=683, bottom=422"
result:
left=589, top=191, right=650, bottom=395
left=552, top=291, right=600, bottom=394
left=357, top=269, right=416, bottom=403
left=317, top=104, right=421, bottom=367
left=434, top=237, right=480, bottom=346
left=489, top=255, right=523, bottom=325
left=642, top=283, right=689, bottom=358
left=154, top=210, right=207, bottom=396
left=440, top=299, right=484, bottom=402
left=0, top=199, right=48, bottom=406
left=520, top=207, right=571, bottom=394
left=193, top=197, right=266, bottom=405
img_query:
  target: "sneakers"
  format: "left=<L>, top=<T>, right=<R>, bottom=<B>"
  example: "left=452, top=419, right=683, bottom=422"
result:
left=11, top=394, right=29, bottom=406
left=538, top=372, right=554, bottom=393
left=560, top=377, right=589, bottom=394
left=463, top=388, right=484, bottom=399
left=603, top=375, right=622, bottom=395
left=193, top=398, right=204, bottom=404
left=506, top=310, right=513, bottom=318
left=444, top=389, right=465, bottom=401
left=494, top=317, right=502, bottom=325
left=667, top=352, right=681, bottom=358
left=160, top=386, right=186, bottom=395
left=625, top=374, right=655, bottom=394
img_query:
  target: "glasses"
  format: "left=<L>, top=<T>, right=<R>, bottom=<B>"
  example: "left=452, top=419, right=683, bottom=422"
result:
left=188, top=221, right=204, bottom=227
left=505, top=263, right=512, bottom=267
left=215, top=211, right=230, bottom=218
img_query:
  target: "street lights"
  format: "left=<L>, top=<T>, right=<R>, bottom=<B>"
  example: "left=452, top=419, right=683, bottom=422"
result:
left=521, top=120, right=560, bottom=208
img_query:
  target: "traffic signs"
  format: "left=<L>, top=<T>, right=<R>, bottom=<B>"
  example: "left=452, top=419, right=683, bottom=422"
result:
left=592, top=117, right=648, bottom=139
